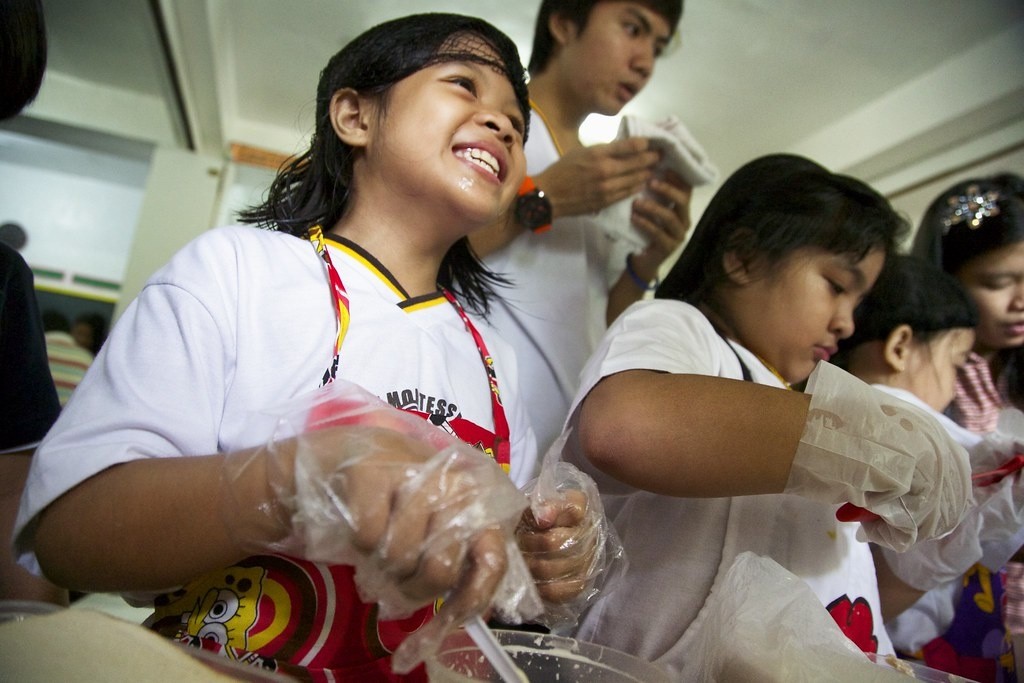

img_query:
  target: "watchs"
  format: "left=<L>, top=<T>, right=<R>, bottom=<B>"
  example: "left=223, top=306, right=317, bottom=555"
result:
left=515, top=178, right=553, bottom=234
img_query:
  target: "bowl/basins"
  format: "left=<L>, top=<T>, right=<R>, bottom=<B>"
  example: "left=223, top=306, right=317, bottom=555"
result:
left=427, top=629, right=674, bottom=683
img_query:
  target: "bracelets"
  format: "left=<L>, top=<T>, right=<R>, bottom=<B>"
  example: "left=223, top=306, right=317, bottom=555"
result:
left=627, top=253, right=658, bottom=290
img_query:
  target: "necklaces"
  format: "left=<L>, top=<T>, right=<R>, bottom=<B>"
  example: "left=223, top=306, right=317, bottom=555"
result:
left=527, top=97, right=566, bottom=158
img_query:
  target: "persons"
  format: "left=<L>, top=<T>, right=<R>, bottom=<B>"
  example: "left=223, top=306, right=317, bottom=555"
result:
left=10, top=14, right=610, bottom=683
left=543, top=153, right=1024, bottom=683
left=448, top=0, right=691, bottom=464
left=0, top=0, right=108, bottom=606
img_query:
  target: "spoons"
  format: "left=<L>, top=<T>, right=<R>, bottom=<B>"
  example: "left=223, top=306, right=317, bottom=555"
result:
left=836, top=455, right=1024, bottom=522
